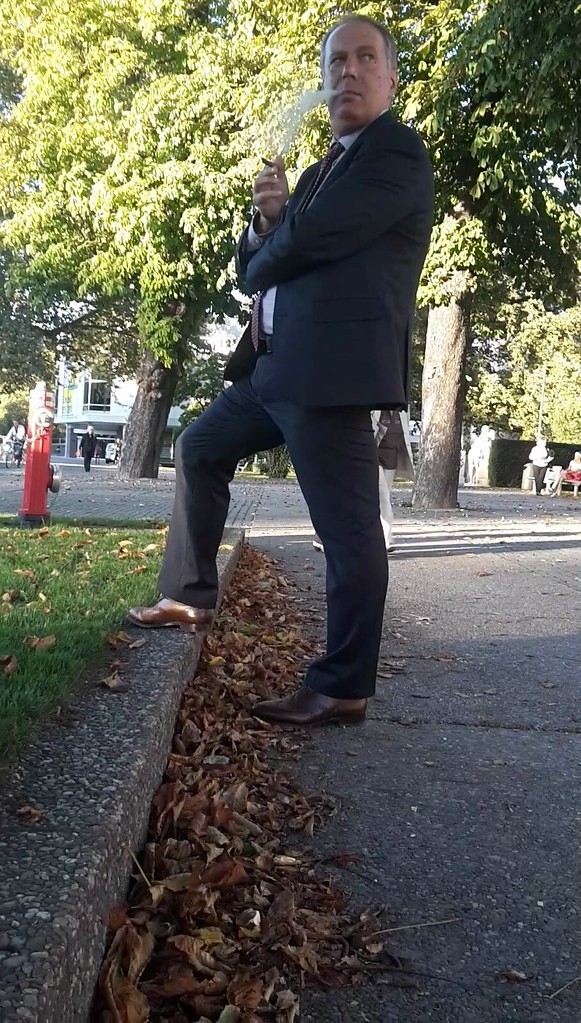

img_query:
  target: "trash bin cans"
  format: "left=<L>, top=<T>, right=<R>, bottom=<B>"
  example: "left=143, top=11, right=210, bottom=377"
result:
left=520, top=463, right=535, bottom=490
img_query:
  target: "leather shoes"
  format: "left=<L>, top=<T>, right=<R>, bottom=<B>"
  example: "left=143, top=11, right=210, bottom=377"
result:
left=125, top=596, right=214, bottom=633
left=251, top=682, right=367, bottom=726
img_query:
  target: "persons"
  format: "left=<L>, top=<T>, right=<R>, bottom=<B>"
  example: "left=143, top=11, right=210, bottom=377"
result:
left=4, top=420, right=27, bottom=467
left=118, top=13, right=441, bottom=725
left=526, top=436, right=581, bottom=499
left=79, top=425, right=101, bottom=471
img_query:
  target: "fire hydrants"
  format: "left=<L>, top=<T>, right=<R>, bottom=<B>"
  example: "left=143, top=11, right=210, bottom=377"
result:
left=16, top=379, right=64, bottom=527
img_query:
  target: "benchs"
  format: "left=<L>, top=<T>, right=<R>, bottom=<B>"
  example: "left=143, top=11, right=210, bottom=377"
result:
left=528, top=466, right=581, bottom=499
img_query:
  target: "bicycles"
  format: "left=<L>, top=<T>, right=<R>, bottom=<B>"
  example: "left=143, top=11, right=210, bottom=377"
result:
left=5, top=440, right=24, bottom=468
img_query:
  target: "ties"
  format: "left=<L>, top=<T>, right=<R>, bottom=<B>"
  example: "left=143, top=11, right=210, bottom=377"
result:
left=250, top=142, right=347, bottom=353
